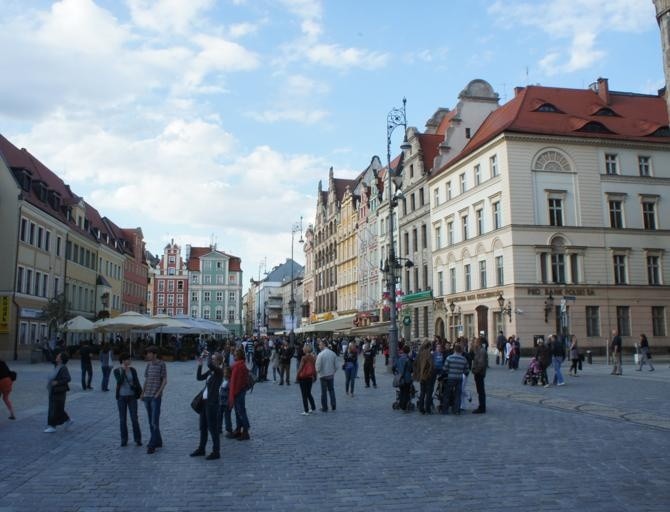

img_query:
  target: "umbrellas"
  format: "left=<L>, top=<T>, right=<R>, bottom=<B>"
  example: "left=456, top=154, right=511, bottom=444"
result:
left=57, top=310, right=230, bottom=360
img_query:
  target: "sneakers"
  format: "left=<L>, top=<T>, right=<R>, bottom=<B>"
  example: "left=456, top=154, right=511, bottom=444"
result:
left=148, top=447, right=155, bottom=453
left=189, top=428, right=249, bottom=460
left=42, top=427, right=56, bottom=433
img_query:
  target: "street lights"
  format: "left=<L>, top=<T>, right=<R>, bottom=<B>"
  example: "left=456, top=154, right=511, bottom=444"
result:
left=256, top=254, right=270, bottom=337
left=289, top=215, right=307, bottom=346
left=100, top=292, right=111, bottom=345
left=379, top=95, right=414, bottom=371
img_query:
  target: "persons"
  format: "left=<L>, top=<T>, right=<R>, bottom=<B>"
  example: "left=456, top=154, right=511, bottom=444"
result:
left=76, top=340, right=95, bottom=390
left=234, top=332, right=390, bottom=388
left=43, top=352, right=74, bottom=432
left=345, top=342, right=358, bottom=397
left=610, top=330, right=622, bottom=375
left=535, top=333, right=580, bottom=387
left=35, top=333, right=229, bottom=362
left=0, top=360, right=16, bottom=420
left=97, top=342, right=114, bottom=391
left=141, top=345, right=167, bottom=454
left=296, top=344, right=317, bottom=415
left=190, top=349, right=250, bottom=459
left=113, top=353, right=143, bottom=447
left=394, top=336, right=488, bottom=414
left=315, top=339, right=339, bottom=412
left=496, top=331, right=520, bottom=371
left=635, top=334, right=655, bottom=371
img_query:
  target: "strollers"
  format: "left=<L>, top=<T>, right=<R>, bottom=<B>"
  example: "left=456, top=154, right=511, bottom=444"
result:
left=390, top=381, right=419, bottom=412
left=432, top=376, right=447, bottom=411
left=520, top=356, right=541, bottom=386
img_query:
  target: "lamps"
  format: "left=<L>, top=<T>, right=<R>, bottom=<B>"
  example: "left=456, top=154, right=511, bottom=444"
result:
left=497, top=293, right=511, bottom=322
left=544, top=290, right=553, bottom=322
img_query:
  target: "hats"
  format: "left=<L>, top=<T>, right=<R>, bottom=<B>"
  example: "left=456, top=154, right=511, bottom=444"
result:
left=144, top=345, right=161, bottom=355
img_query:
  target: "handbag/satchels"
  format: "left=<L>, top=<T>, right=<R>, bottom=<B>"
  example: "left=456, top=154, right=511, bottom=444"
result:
left=244, top=371, right=256, bottom=388
left=191, top=394, right=204, bottom=414
left=108, top=357, right=113, bottom=368
left=392, top=374, right=405, bottom=387
left=132, top=383, right=141, bottom=399
left=299, top=362, right=315, bottom=379
